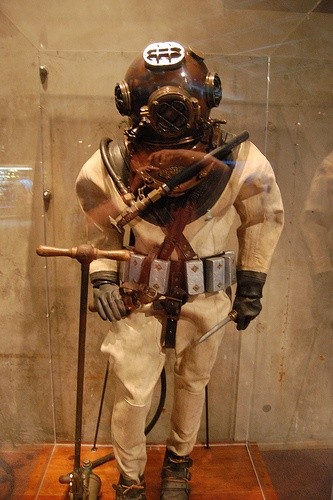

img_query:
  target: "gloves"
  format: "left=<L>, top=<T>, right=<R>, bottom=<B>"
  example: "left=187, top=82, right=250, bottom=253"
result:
left=90, top=271, right=127, bottom=322
left=232, top=270, right=267, bottom=330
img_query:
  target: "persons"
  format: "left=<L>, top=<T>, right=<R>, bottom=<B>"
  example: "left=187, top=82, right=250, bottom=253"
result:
left=74, top=53, right=285, bottom=500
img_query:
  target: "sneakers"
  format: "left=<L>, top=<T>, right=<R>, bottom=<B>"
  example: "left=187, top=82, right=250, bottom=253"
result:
left=160, top=447, right=192, bottom=500
left=112, top=474, right=147, bottom=500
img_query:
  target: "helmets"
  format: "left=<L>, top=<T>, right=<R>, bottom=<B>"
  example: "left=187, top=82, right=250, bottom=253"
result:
left=115, top=42, right=223, bottom=146
